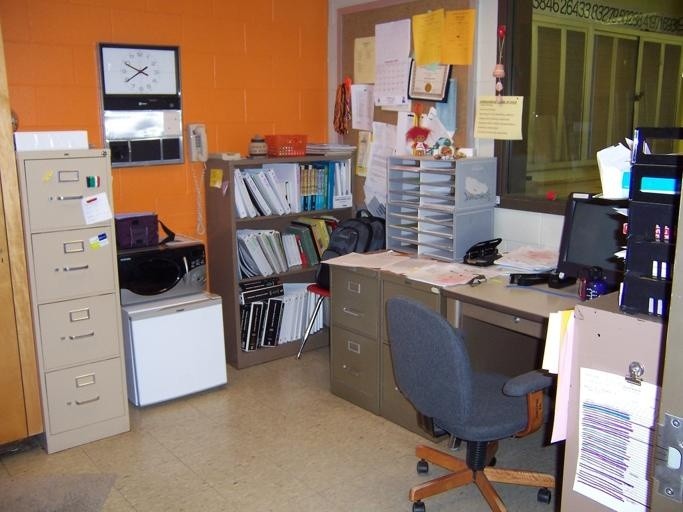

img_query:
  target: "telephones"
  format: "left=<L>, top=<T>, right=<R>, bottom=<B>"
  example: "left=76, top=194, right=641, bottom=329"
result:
left=188, top=124, right=209, bottom=162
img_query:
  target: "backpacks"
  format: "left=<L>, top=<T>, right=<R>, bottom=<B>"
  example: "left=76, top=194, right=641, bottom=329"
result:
left=312, top=207, right=387, bottom=294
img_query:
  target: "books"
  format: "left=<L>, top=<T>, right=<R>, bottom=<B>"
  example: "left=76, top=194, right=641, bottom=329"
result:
left=300, top=159, right=352, bottom=211
left=281, top=215, right=340, bottom=267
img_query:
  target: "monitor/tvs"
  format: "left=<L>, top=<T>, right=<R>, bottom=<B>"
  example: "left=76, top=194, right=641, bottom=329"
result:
left=556, top=191, right=629, bottom=285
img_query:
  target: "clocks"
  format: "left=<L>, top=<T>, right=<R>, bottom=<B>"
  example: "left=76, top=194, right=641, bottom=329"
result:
left=96, top=39, right=183, bottom=115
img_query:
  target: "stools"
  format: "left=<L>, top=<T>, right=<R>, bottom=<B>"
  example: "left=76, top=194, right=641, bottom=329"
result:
left=294, top=282, right=330, bottom=359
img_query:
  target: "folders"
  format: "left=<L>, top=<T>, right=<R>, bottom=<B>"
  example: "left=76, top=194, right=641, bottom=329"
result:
left=233, top=169, right=292, bottom=219
left=237, top=228, right=288, bottom=278
left=276, top=281, right=328, bottom=346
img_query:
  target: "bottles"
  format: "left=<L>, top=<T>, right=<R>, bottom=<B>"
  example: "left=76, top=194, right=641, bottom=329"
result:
left=578, top=265, right=607, bottom=302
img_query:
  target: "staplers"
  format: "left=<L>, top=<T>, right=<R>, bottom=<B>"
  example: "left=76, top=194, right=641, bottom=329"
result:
left=509, top=273, right=554, bottom=286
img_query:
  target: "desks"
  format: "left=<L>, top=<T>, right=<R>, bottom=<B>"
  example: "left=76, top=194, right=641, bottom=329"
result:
left=321, top=246, right=586, bottom=447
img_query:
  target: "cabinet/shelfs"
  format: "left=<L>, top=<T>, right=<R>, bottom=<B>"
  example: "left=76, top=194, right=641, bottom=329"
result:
left=202, top=150, right=360, bottom=372
left=14, top=147, right=133, bottom=457
left=384, top=156, right=497, bottom=264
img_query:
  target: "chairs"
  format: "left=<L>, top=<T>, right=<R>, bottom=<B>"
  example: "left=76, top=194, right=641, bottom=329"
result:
left=382, top=292, right=560, bottom=512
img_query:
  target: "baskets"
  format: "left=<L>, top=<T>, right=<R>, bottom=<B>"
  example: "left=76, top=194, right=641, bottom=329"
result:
left=265, top=134, right=308, bottom=159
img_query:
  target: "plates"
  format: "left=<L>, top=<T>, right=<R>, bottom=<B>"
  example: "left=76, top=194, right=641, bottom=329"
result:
left=305, top=143, right=357, bottom=155
